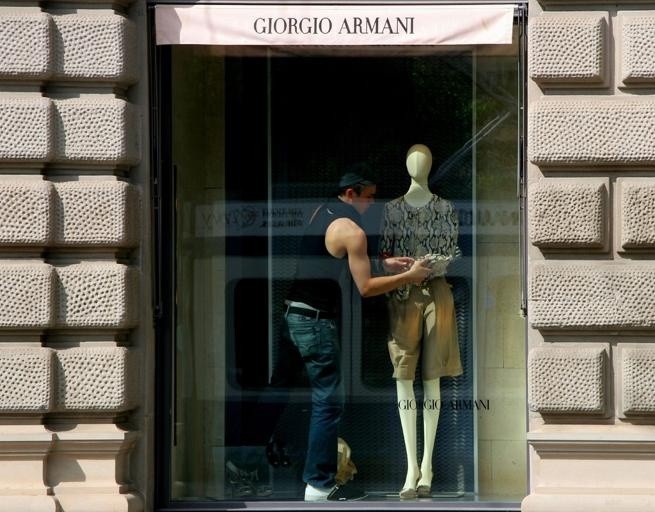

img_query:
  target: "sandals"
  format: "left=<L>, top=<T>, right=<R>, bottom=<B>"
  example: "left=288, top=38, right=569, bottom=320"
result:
left=229, top=479, right=272, bottom=498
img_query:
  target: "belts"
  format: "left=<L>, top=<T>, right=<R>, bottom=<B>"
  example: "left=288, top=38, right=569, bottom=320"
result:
left=284, top=305, right=333, bottom=319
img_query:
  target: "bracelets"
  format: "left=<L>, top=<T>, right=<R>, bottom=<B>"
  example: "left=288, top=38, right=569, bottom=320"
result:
left=376, top=258, right=386, bottom=274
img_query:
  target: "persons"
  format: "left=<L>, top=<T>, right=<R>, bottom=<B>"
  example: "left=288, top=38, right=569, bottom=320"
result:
left=277, top=166, right=437, bottom=502
left=374, top=141, right=463, bottom=502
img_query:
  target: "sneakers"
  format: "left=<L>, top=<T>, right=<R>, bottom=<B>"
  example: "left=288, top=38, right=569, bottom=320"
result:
left=304, top=483, right=368, bottom=500
